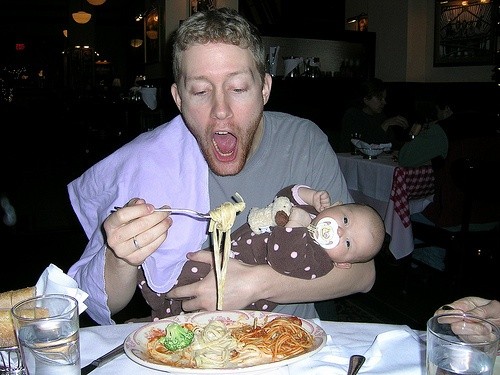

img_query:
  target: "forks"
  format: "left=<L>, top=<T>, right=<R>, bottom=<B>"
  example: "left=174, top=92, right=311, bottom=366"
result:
left=112, top=205, right=212, bottom=219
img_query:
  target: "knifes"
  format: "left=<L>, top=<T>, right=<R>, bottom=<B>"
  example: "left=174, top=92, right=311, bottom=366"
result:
left=80, top=344, right=125, bottom=375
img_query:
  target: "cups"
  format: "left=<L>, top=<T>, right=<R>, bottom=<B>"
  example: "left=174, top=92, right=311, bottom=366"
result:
left=10, top=293, right=81, bottom=375
left=425, top=313, right=500, bottom=375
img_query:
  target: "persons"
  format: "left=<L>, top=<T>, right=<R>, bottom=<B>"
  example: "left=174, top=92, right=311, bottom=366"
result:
left=398, top=93, right=474, bottom=225
left=66, top=7, right=376, bottom=325
left=139, top=183, right=385, bottom=326
left=434, top=296, right=500, bottom=351
left=339, top=76, right=408, bottom=153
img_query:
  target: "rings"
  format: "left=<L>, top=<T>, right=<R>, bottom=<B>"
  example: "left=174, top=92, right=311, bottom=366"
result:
left=133, top=238, right=140, bottom=249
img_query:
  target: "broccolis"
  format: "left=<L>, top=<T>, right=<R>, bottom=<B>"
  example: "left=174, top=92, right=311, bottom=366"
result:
left=160, top=323, right=195, bottom=351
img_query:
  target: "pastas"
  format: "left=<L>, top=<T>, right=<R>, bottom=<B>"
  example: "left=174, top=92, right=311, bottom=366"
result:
left=232, top=317, right=314, bottom=361
left=209, top=191, right=245, bottom=311
left=187, top=320, right=271, bottom=367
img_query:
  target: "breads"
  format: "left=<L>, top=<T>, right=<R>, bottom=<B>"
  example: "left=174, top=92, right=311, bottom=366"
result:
left=0, top=286, right=48, bottom=348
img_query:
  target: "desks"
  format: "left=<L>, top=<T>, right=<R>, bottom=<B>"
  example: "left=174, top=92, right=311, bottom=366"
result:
left=335, top=151, right=436, bottom=260
left=23, top=317, right=427, bottom=375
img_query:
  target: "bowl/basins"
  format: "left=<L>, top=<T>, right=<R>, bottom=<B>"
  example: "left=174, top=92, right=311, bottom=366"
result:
left=356, top=147, right=385, bottom=160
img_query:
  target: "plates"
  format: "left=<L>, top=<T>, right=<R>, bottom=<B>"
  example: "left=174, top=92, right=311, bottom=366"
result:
left=123, top=310, right=327, bottom=375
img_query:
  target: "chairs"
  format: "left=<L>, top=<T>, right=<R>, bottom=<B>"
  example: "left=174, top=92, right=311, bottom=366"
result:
left=412, top=163, right=485, bottom=268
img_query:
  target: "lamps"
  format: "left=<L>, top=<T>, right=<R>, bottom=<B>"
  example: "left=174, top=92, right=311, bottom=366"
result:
left=72, top=9, right=91, bottom=24
left=86, top=0, right=105, bottom=6
left=348, top=16, right=358, bottom=24
